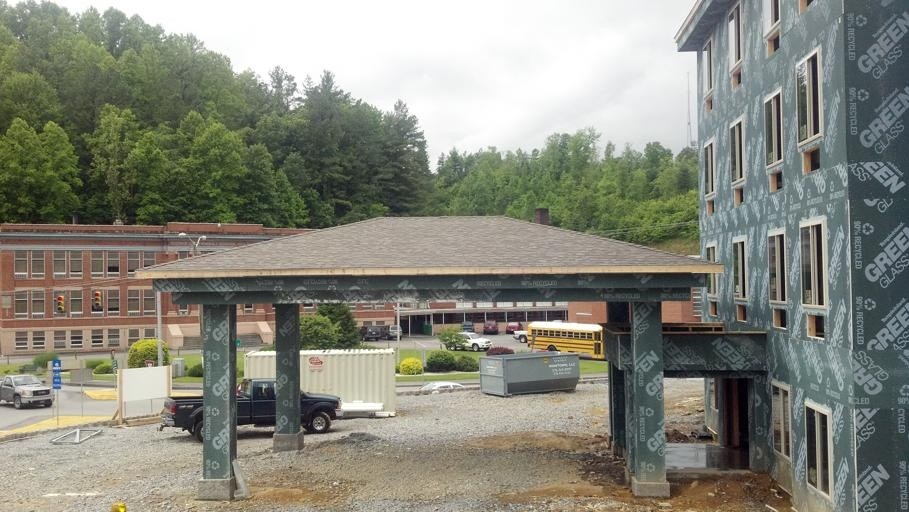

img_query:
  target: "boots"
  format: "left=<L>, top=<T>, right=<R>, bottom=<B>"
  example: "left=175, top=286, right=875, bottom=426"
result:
left=381, top=324, right=403, bottom=340
left=483, top=320, right=498, bottom=335
left=460, top=321, right=474, bottom=332
left=359, top=325, right=381, bottom=342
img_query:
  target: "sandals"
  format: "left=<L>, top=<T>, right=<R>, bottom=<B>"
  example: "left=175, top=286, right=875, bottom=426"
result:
left=526, top=321, right=604, bottom=359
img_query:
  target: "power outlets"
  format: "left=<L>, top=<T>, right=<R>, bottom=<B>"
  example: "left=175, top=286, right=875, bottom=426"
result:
left=514, top=330, right=528, bottom=343
left=161, top=377, right=342, bottom=442
left=0, top=373, right=55, bottom=409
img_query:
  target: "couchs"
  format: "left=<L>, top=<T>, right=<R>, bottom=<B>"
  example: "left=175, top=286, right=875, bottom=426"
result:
left=57, top=296, right=64, bottom=313
left=94, top=291, right=101, bottom=307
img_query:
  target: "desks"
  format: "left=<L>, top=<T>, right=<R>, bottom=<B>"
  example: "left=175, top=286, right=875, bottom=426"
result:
left=179, top=231, right=207, bottom=368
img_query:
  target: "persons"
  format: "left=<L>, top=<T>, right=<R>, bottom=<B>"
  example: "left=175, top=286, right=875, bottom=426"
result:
left=527, top=343, right=532, bottom=353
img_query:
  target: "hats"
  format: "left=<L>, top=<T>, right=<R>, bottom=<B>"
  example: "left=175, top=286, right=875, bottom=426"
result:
left=505, top=321, right=523, bottom=333
left=447, top=332, right=492, bottom=352
